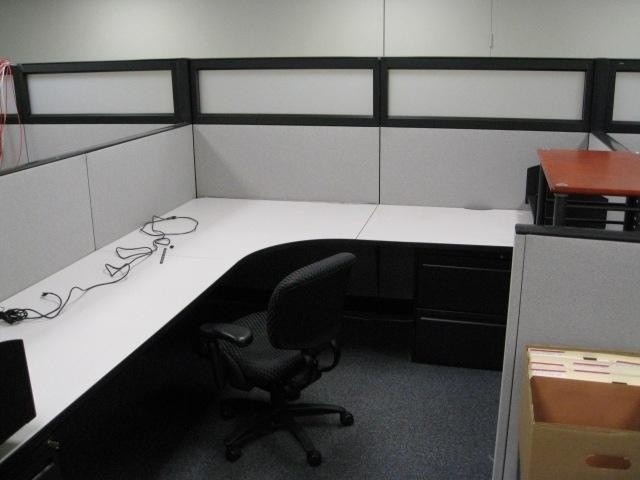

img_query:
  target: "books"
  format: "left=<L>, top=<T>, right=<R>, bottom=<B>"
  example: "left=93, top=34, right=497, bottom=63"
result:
left=526, top=346, right=640, bottom=432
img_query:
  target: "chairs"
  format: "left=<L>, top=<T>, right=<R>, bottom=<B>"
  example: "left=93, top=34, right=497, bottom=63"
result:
left=182, top=247, right=364, bottom=467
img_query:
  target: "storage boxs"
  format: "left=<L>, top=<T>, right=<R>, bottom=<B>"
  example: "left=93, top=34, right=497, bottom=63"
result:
left=519, top=342, right=640, bottom=479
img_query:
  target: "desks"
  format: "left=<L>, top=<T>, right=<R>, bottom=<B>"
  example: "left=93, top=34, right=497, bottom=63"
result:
left=0, top=194, right=531, bottom=479
